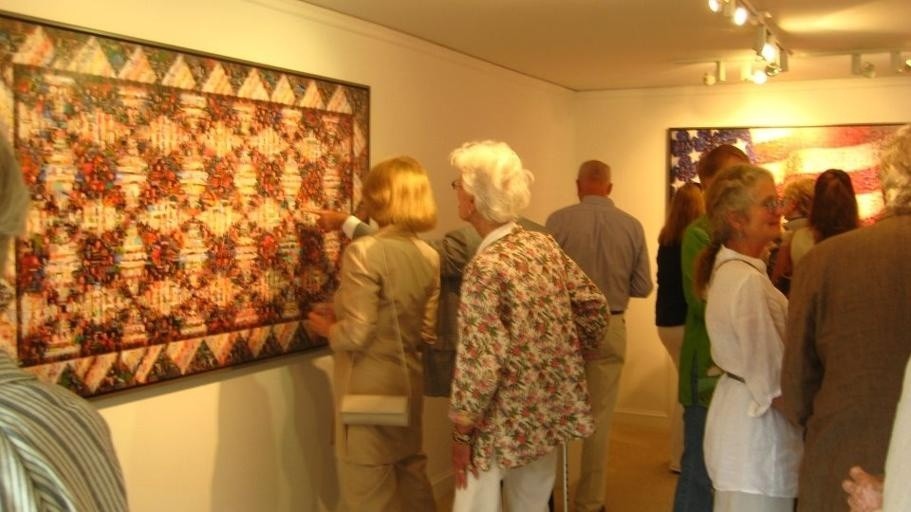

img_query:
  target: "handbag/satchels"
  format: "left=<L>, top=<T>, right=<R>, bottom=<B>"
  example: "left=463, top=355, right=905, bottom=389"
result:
left=340, top=393, right=410, bottom=427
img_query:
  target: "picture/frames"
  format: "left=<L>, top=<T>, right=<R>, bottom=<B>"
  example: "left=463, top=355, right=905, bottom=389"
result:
left=0, top=8, right=375, bottom=402
left=663, top=125, right=909, bottom=231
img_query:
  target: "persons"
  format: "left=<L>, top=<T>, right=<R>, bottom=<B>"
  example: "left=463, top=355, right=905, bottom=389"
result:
left=309, top=138, right=610, bottom=512
left=546, top=160, right=651, bottom=512
left=655, top=125, right=911, bottom=512
left=0, top=130, right=127, bottom=512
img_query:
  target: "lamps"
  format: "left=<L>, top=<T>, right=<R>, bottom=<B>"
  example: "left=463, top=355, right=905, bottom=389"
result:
left=699, top=0, right=911, bottom=88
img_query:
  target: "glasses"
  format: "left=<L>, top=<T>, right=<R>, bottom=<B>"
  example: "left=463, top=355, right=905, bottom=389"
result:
left=758, top=193, right=787, bottom=215
